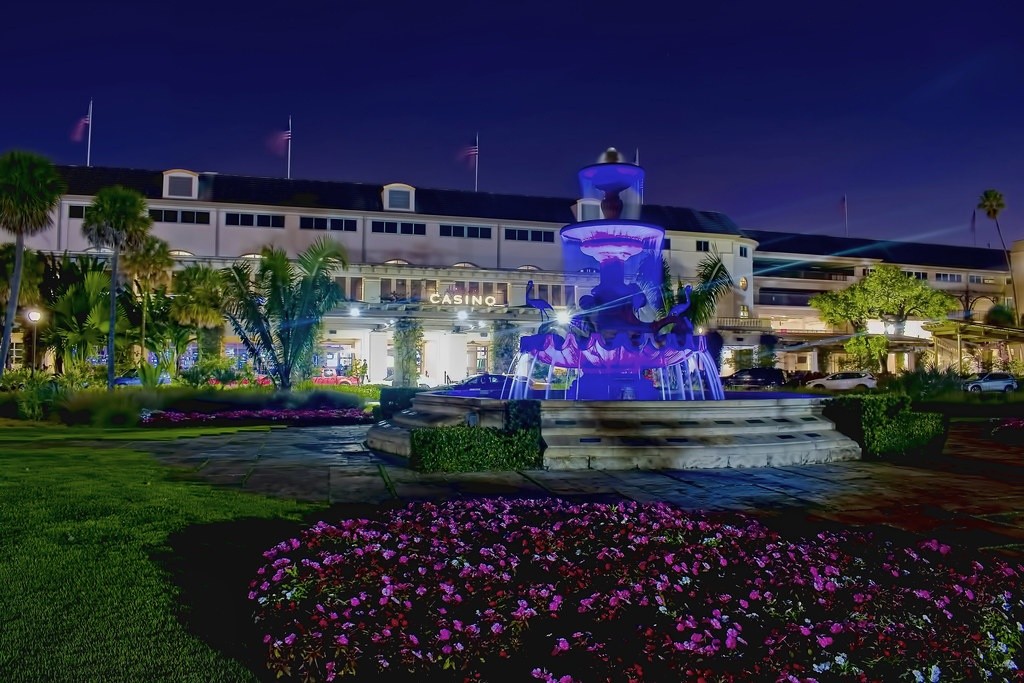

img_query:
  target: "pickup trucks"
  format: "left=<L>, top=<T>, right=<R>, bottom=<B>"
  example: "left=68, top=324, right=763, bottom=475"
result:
left=312, top=367, right=359, bottom=386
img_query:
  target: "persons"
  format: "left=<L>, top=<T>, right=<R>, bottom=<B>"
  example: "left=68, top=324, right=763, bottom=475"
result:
left=786, top=370, right=824, bottom=385
left=361, top=359, right=371, bottom=384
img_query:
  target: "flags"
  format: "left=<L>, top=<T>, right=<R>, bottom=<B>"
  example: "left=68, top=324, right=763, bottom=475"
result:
left=454, top=135, right=478, bottom=165
left=838, top=197, right=847, bottom=215
left=71, top=110, right=90, bottom=142
left=267, top=127, right=291, bottom=155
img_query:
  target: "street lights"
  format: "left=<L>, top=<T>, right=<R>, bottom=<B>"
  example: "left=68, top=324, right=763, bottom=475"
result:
left=28, top=311, right=41, bottom=379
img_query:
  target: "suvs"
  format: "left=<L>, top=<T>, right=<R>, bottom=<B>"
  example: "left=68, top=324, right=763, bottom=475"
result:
left=963, top=372, right=1017, bottom=394
left=718, top=368, right=788, bottom=391
left=806, top=371, right=880, bottom=390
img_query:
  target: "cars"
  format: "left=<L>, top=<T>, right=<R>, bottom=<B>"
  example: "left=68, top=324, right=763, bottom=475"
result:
left=789, top=370, right=829, bottom=387
left=446, top=373, right=534, bottom=398
left=113, top=369, right=172, bottom=386
left=209, top=374, right=271, bottom=386
